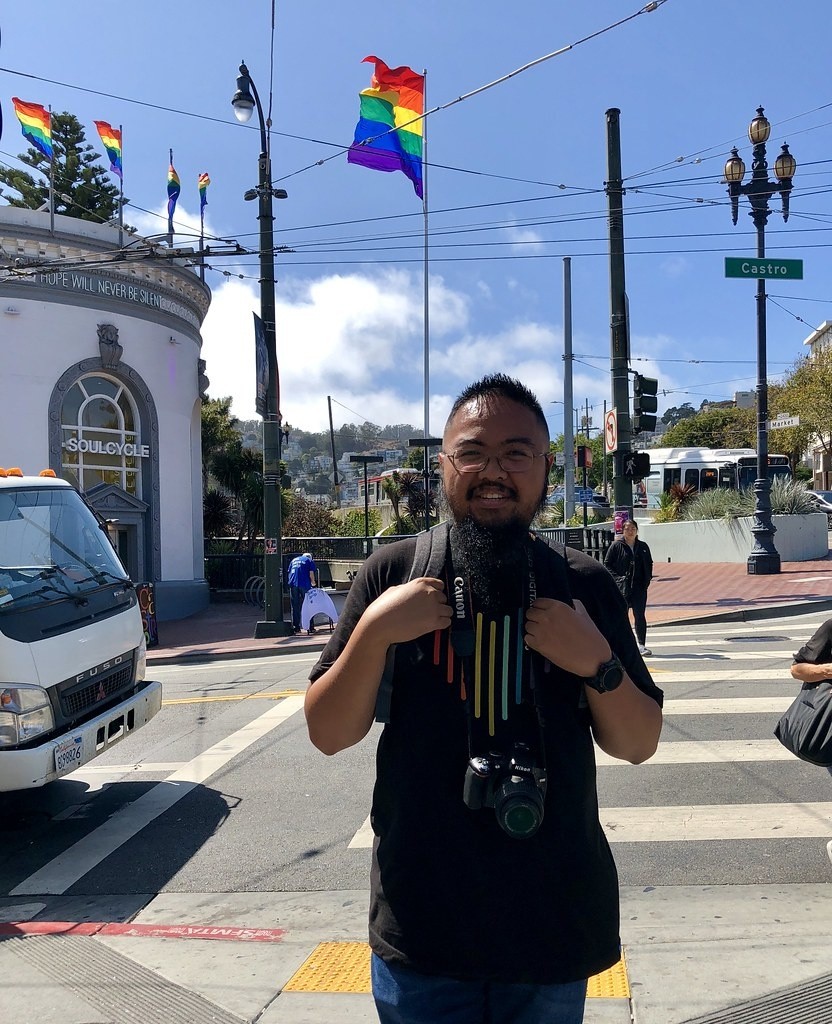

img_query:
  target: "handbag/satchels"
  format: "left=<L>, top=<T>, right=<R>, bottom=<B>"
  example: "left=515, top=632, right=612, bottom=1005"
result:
left=773, top=682, right=832, bottom=767
left=615, top=575, right=631, bottom=597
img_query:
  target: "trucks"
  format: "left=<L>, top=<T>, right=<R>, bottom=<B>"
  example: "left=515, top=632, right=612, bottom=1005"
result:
left=0, top=467, right=162, bottom=794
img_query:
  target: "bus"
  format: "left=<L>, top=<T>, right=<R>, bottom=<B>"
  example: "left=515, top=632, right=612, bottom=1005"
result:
left=633, top=446, right=794, bottom=510
left=359, top=468, right=438, bottom=506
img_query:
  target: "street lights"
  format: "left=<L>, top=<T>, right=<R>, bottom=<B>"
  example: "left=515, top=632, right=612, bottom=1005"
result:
left=231, top=58, right=292, bottom=637
left=724, top=105, right=797, bottom=575
left=551, top=401, right=579, bottom=484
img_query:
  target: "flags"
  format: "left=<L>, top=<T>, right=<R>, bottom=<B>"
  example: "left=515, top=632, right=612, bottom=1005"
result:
left=11, top=96, right=54, bottom=161
left=198, top=173, right=211, bottom=219
left=94, top=121, right=123, bottom=180
left=347, top=56, right=424, bottom=196
left=166, top=164, right=180, bottom=233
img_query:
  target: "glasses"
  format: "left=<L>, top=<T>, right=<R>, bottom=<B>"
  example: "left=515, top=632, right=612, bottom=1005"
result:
left=443, top=446, right=550, bottom=473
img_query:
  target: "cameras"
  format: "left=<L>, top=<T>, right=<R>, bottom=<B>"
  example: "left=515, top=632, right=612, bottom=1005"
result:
left=463, top=742, right=548, bottom=839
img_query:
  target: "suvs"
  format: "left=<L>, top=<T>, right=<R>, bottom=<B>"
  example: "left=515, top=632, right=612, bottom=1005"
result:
left=549, top=485, right=610, bottom=507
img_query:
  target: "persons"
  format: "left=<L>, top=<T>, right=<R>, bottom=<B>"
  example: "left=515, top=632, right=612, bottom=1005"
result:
left=287, top=552, right=317, bottom=633
left=791, top=617, right=831, bottom=871
left=303, top=373, right=663, bottom=1023
left=603, top=519, right=653, bottom=656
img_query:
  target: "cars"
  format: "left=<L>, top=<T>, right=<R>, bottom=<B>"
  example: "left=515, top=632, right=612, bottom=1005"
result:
left=546, top=493, right=602, bottom=508
left=803, top=490, right=832, bottom=530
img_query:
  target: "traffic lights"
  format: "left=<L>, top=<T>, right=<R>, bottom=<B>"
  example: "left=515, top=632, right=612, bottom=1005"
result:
left=633, top=375, right=658, bottom=434
left=622, top=453, right=650, bottom=480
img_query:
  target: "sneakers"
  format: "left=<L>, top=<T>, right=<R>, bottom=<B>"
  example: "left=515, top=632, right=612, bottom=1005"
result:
left=638, top=645, right=652, bottom=656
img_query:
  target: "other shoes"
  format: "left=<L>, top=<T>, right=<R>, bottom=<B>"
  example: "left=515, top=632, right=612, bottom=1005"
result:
left=826, top=840, right=832, bottom=863
